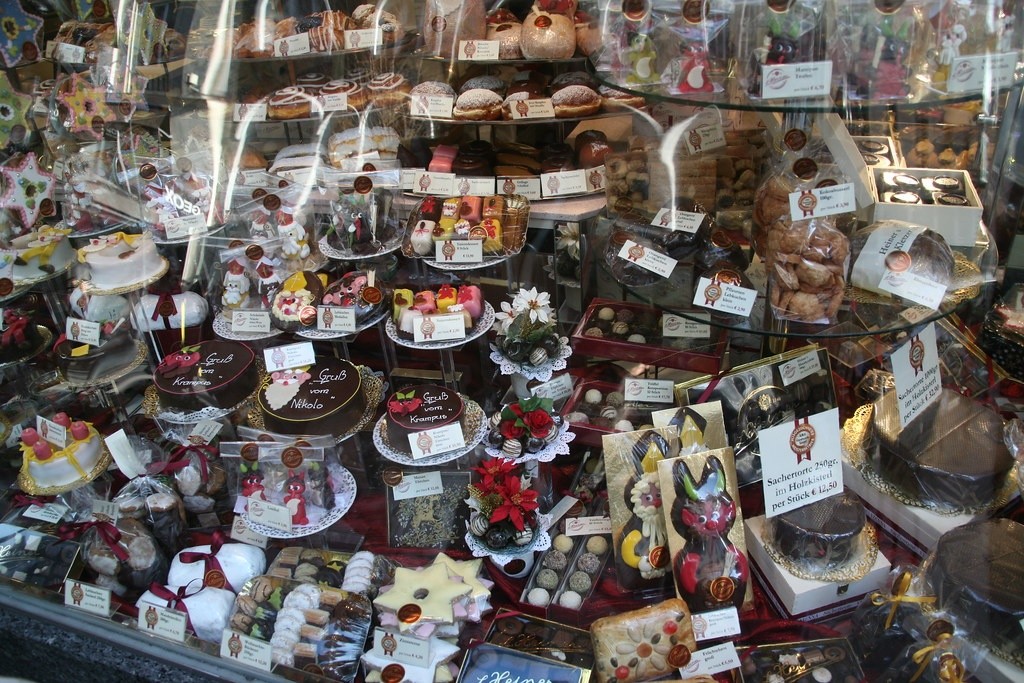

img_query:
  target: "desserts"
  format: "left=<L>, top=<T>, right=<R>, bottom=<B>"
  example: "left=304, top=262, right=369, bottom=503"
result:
left=1, top=528, right=602, bottom=683
left=530, top=0, right=1024, bottom=683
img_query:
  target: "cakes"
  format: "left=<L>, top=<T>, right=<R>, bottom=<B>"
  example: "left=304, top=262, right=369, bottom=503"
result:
left=0, top=182, right=513, bottom=547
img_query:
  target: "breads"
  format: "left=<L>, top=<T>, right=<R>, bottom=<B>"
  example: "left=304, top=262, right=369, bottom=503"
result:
left=148, top=0, right=654, bottom=196
